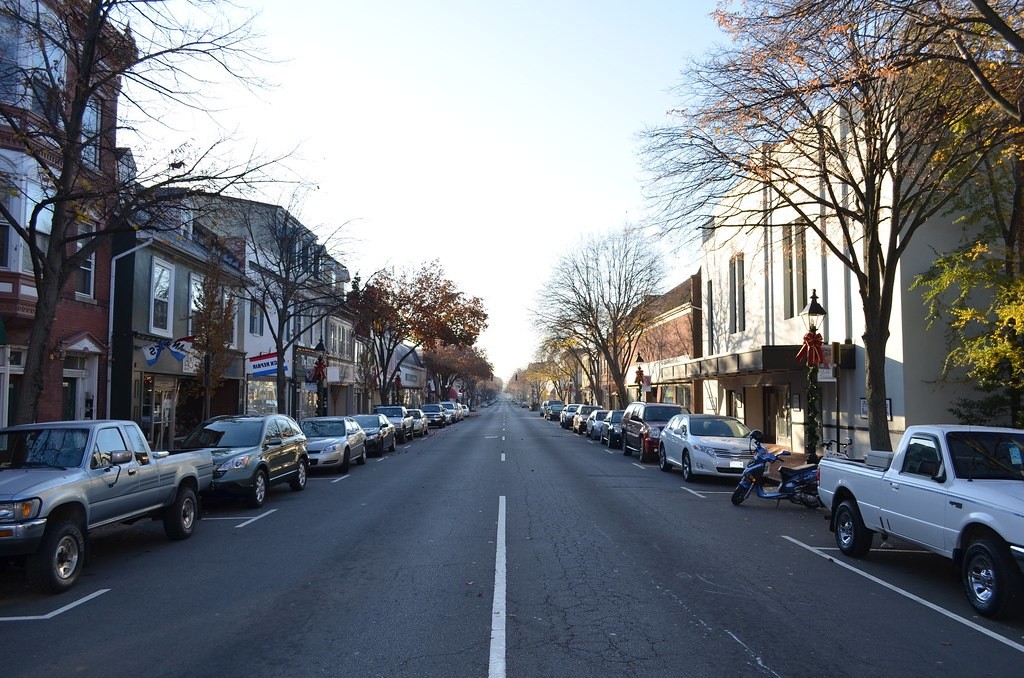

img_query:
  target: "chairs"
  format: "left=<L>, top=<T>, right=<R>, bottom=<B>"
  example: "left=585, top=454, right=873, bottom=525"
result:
left=687, top=421, right=703, bottom=426
left=39, top=435, right=56, bottom=460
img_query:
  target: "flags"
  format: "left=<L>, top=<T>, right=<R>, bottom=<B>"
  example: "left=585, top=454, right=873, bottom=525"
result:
left=250, top=354, right=291, bottom=378
left=141, top=335, right=196, bottom=367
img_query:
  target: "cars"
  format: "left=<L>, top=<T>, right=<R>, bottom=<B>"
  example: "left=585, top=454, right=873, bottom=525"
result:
left=599, top=410, right=627, bottom=450
left=457, top=402, right=464, bottom=422
left=462, top=405, right=470, bottom=417
left=443, top=408, right=453, bottom=426
left=406, top=409, right=429, bottom=437
left=349, top=414, right=396, bottom=458
left=586, top=410, right=610, bottom=441
left=480, top=397, right=499, bottom=408
left=540, top=401, right=585, bottom=430
left=299, top=416, right=367, bottom=476
left=181, top=414, right=309, bottom=509
left=510, top=398, right=537, bottom=412
left=658, top=414, right=770, bottom=484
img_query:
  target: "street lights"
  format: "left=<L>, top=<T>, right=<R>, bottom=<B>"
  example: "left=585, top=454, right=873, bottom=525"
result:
left=395, top=366, right=403, bottom=406
left=567, top=379, right=573, bottom=404
left=314, top=334, right=328, bottom=416
left=633, top=352, right=645, bottom=402
left=799, top=288, right=829, bottom=466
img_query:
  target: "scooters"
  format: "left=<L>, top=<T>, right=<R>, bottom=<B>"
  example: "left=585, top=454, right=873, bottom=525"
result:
left=731, top=429, right=821, bottom=511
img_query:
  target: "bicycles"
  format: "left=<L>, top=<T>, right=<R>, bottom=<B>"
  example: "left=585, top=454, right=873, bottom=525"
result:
left=819, top=435, right=853, bottom=459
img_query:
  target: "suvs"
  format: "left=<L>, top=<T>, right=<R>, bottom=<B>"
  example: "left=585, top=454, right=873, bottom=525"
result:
left=573, top=405, right=605, bottom=435
left=371, top=404, right=415, bottom=444
left=621, top=400, right=693, bottom=463
left=440, top=402, right=459, bottom=423
left=422, top=404, right=445, bottom=429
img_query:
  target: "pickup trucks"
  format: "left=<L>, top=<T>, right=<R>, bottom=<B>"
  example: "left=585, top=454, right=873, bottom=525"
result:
left=0, top=421, right=213, bottom=596
left=817, top=425, right=1024, bottom=622
